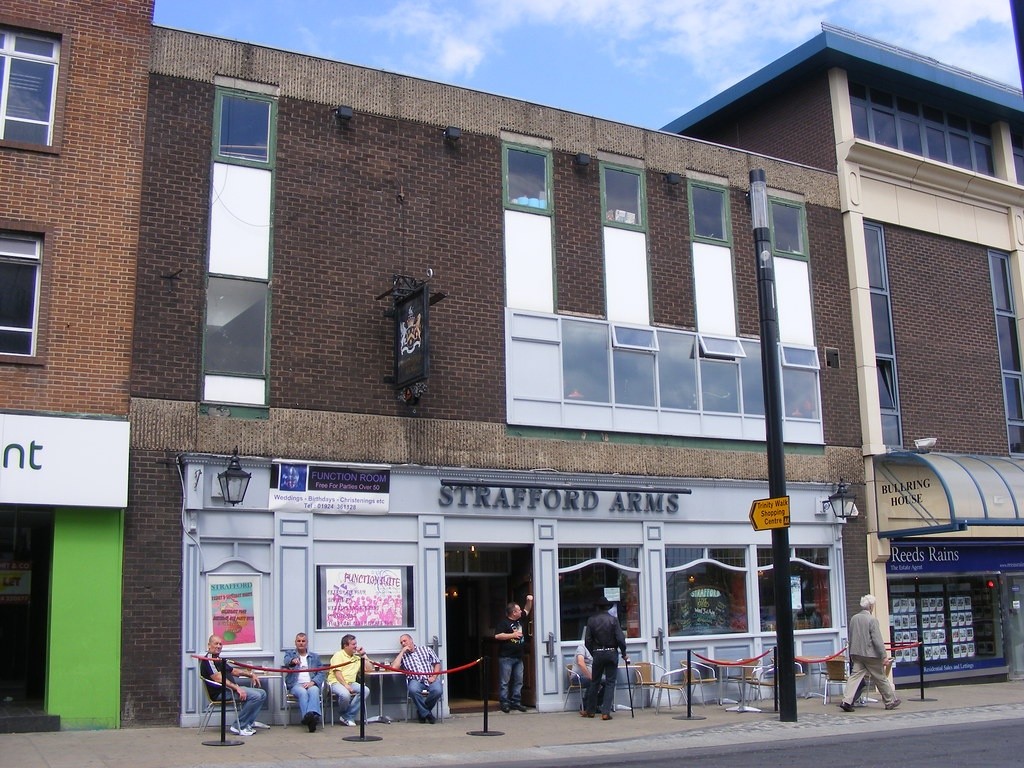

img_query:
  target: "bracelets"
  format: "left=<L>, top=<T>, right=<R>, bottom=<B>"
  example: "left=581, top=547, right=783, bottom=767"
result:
left=361, top=652, right=366, bottom=656
left=249, top=672, right=253, bottom=677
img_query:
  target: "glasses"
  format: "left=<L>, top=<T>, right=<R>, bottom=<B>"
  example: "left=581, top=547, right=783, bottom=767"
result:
left=296, top=640, right=307, bottom=643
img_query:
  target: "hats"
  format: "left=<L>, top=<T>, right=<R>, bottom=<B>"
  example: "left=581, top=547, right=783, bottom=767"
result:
left=593, top=597, right=614, bottom=609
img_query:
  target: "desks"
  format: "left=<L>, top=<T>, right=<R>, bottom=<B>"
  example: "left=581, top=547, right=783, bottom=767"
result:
left=701, top=659, right=733, bottom=708
left=726, top=665, right=762, bottom=713
left=797, top=655, right=828, bottom=700
left=239, top=675, right=283, bottom=729
left=365, top=672, right=405, bottom=723
left=612, top=665, right=641, bottom=712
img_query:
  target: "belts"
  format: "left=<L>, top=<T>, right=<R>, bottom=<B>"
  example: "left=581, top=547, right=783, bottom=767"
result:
left=592, top=648, right=616, bottom=651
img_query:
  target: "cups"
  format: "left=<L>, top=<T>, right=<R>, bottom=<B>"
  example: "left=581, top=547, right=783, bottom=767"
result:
left=262, top=661, right=268, bottom=675
left=374, top=662, right=380, bottom=671
left=626, top=658, right=630, bottom=666
left=384, top=660, right=390, bottom=671
left=246, top=661, right=252, bottom=672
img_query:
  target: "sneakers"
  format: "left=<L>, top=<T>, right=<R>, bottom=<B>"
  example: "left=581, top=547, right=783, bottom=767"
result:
left=230, top=726, right=253, bottom=736
left=247, top=724, right=257, bottom=734
left=340, top=715, right=357, bottom=727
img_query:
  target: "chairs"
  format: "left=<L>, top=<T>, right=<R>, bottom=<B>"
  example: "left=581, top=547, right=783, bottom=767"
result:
left=563, top=664, right=602, bottom=712
left=633, top=654, right=896, bottom=715
left=405, top=674, right=444, bottom=723
left=283, top=675, right=325, bottom=729
left=327, top=675, right=368, bottom=726
left=197, top=676, right=245, bottom=735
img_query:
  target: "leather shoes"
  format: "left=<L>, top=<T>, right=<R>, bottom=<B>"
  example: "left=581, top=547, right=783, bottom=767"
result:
left=885, top=699, right=901, bottom=710
left=839, top=702, right=855, bottom=712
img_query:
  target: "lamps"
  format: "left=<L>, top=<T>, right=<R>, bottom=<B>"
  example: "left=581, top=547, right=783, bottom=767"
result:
left=442, top=126, right=461, bottom=140
left=816, top=477, right=858, bottom=520
left=574, top=153, right=590, bottom=166
left=666, top=173, right=682, bottom=184
left=212, top=445, right=254, bottom=506
left=334, top=105, right=353, bottom=119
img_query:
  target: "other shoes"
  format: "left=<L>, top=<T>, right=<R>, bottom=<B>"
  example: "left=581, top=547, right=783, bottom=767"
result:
left=601, top=714, right=614, bottom=720
left=426, top=712, right=435, bottom=724
left=583, top=712, right=596, bottom=718
left=511, top=704, right=527, bottom=711
left=308, top=714, right=320, bottom=733
left=416, top=710, right=426, bottom=724
left=580, top=702, right=603, bottom=714
left=500, top=706, right=510, bottom=713
left=301, top=711, right=314, bottom=725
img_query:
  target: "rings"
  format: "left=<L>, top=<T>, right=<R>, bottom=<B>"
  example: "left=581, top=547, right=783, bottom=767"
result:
left=295, top=660, right=297, bottom=662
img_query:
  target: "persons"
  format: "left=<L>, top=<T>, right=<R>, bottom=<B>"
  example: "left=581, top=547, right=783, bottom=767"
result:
left=390, top=634, right=443, bottom=724
left=327, top=634, right=373, bottom=726
left=201, top=635, right=266, bottom=736
left=494, top=594, right=533, bottom=712
left=839, top=594, right=902, bottom=712
left=283, top=633, right=327, bottom=733
left=571, top=597, right=628, bottom=720
